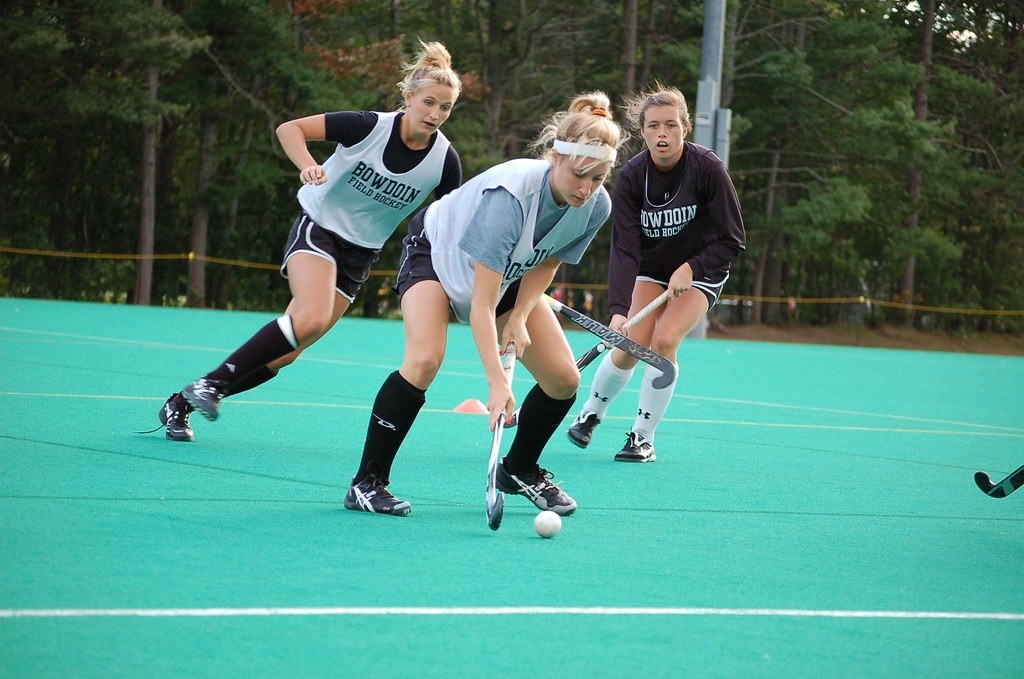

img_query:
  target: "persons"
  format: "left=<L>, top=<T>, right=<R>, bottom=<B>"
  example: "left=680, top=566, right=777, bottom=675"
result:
left=566, top=84, right=747, bottom=464
left=156, top=39, right=464, bottom=442
left=342, top=89, right=621, bottom=519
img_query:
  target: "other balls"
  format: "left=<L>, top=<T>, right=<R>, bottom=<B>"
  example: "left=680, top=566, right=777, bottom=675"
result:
left=534, top=509, right=562, bottom=539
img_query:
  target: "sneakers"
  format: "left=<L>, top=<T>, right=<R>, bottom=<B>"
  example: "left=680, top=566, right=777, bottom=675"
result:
left=343, top=476, right=412, bottom=518
left=614, top=426, right=657, bottom=463
left=496, top=456, right=578, bottom=518
left=134, top=392, right=196, bottom=443
left=567, top=409, right=601, bottom=450
left=181, top=378, right=220, bottom=421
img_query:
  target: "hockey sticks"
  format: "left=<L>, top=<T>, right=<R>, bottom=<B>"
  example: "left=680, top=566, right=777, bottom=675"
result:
left=541, top=293, right=677, bottom=390
left=504, top=287, right=670, bottom=430
left=973, top=465, right=1024, bottom=500
left=484, top=340, right=518, bottom=532
